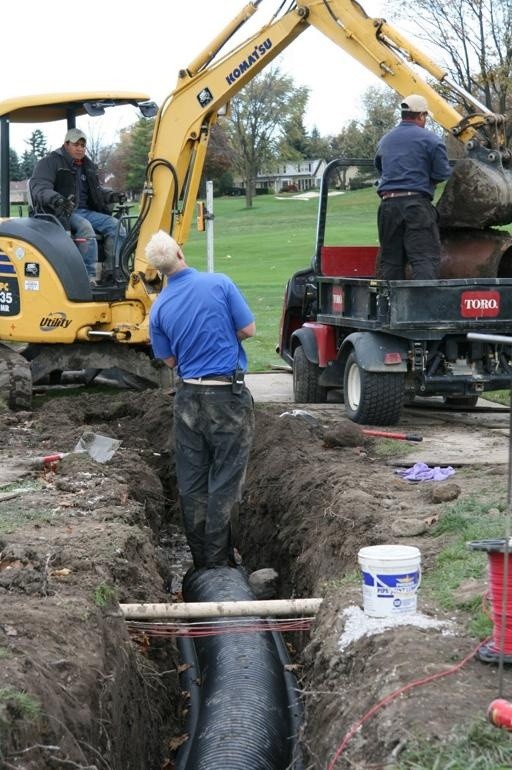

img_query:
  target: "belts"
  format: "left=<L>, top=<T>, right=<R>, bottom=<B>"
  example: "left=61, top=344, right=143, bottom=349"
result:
left=181, top=378, right=234, bottom=386
left=380, top=189, right=422, bottom=200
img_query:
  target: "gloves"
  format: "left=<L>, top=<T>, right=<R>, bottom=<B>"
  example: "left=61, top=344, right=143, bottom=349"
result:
left=59, top=194, right=76, bottom=214
left=109, top=192, right=128, bottom=206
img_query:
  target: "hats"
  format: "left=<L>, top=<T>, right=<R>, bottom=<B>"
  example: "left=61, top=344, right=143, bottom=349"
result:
left=401, top=95, right=433, bottom=116
left=64, top=128, right=88, bottom=144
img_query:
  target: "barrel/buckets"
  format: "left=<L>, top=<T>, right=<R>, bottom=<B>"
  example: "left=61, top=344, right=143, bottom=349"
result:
left=355, top=543, right=422, bottom=619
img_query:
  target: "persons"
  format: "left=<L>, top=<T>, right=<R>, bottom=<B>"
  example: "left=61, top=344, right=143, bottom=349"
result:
left=144, top=229, right=257, bottom=569
left=29, top=128, right=132, bottom=287
left=374, top=94, right=452, bottom=280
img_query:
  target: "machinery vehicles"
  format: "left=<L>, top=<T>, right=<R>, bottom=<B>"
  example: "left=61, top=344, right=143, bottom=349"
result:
left=1, top=0, right=511, bottom=397
left=275, top=159, right=512, bottom=426
